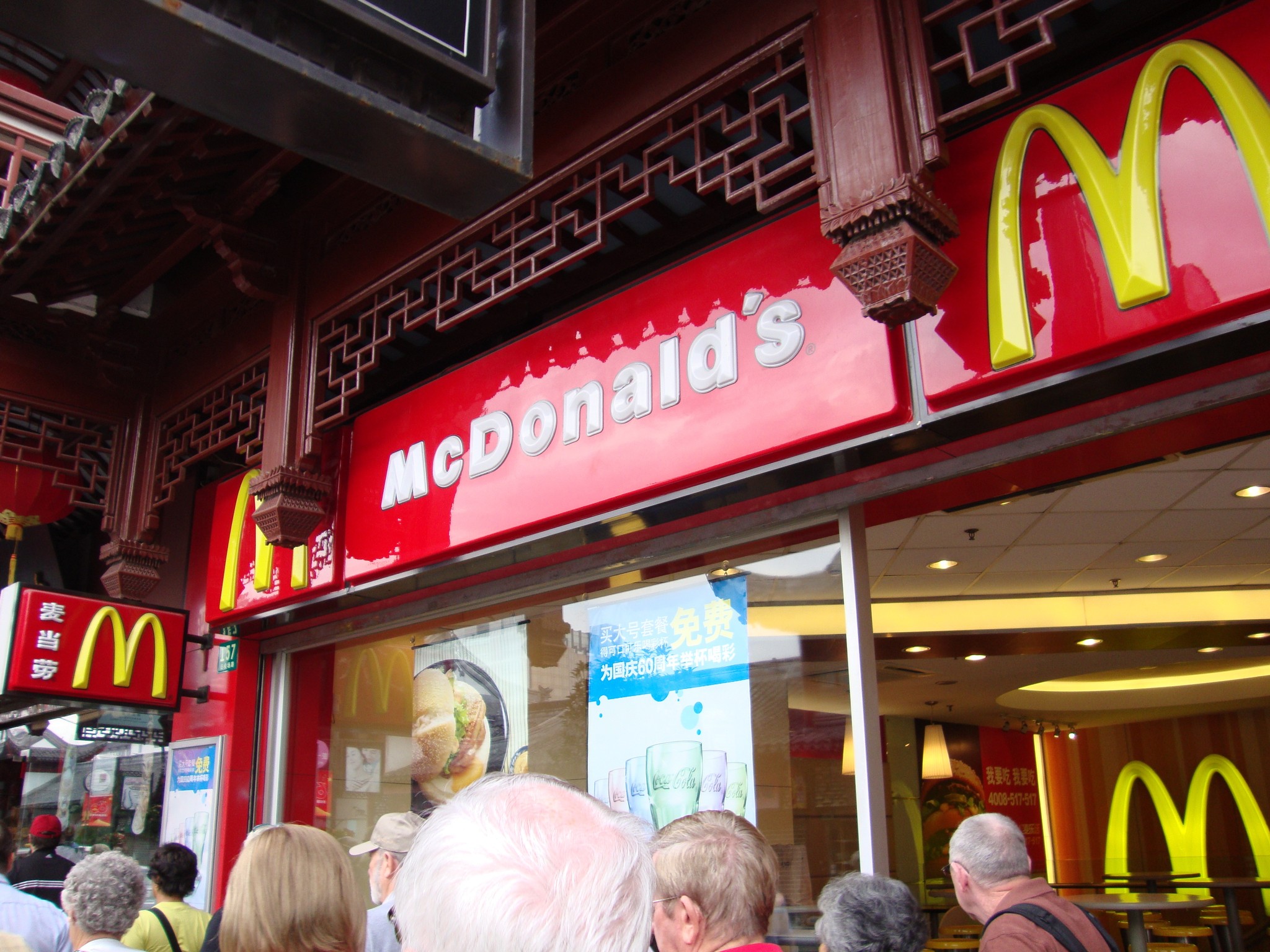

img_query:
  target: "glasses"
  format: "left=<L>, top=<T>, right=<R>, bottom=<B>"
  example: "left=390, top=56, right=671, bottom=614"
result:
left=386, top=906, right=402, bottom=944
left=939, top=861, right=970, bottom=879
left=146, top=870, right=155, bottom=881
left=251, top=822, right=286, bottom=833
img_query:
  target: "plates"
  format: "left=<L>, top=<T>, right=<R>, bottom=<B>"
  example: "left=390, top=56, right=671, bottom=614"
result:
left=410, top=658, right=510, bottom=819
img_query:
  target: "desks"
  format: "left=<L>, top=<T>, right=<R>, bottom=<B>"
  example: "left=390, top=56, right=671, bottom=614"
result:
left=917, top=896, right=964, bottom=939
left=1062, top=892, right=1214, bottom=950
left=1104, top=871, right=1200, bottom=941
left=923, top=877, right=955, bottom=888
left=1168, top=877, right=1270, bottom=952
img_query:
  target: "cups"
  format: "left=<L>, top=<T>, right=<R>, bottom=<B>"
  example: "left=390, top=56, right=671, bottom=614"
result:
left=594, top=740, right=749, bottom=830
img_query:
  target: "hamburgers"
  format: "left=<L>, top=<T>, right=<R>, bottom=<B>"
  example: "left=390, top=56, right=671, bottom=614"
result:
left=411, top=668, right=492, bottom=806
left=920, top=758, right=985, bottom=877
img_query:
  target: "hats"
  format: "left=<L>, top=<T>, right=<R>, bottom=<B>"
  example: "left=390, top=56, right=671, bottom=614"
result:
left=348, top=810, right=428, bottom=857
left=29, top=814, right=62, bottom=839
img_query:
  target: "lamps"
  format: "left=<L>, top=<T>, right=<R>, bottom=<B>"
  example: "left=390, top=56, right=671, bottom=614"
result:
left=1021, top=724, right=1028, bottom=734
left=841, top=716, right=854, bottom=776
left=1003, top=722, right=1011, bottom=731
left=922, top=700, right=952, bottom=779
left=1037, top=726, right=1045, bottom=736
left=1054, top=725, right=1061, bottom=738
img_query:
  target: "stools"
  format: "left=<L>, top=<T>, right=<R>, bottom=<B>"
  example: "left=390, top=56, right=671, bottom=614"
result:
left=924, top=889, right=1254, bottom=950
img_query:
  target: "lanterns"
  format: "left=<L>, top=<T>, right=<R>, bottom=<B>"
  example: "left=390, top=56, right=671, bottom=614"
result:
left=0, top=438, right=84, bottom=586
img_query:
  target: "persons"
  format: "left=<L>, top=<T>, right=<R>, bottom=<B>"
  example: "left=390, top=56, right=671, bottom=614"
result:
left=6, top=813, right=77, bottom=910
left=0, top=827, right=74, bottom=952
left=646, top=809, right=783, bottom=952
left=940, top=812, right=1121, bottom=952
left=61, top=844, right=149, bottom=952
left=813, top=870, right=926, bottom=952
left=392, top=772, right=654, bottom=952
left=199, top=824, right=367, bottom=952
left=119, top=843, right=213, bottom=952
left=347, top=810, right=427, bottom=952
